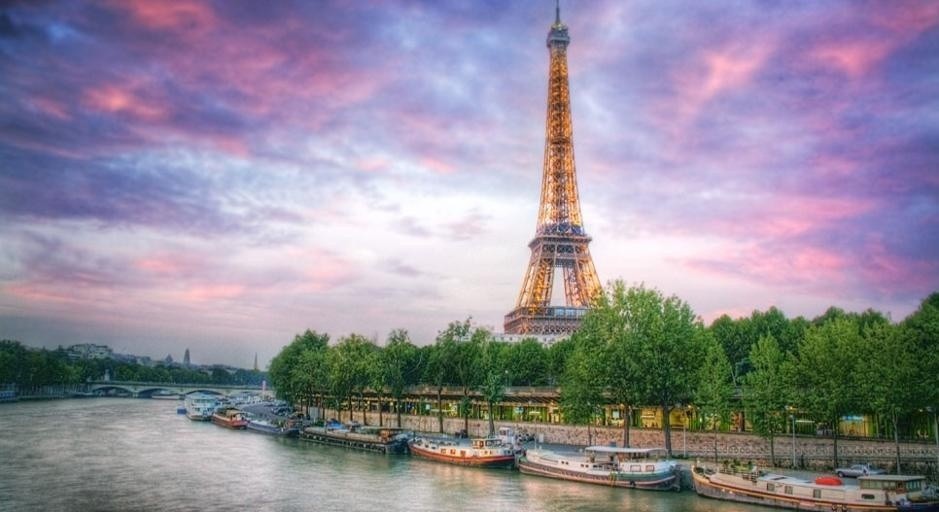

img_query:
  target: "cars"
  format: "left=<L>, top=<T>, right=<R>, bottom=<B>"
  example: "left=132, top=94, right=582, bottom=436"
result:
left=835, top=464, right=883, bottom=478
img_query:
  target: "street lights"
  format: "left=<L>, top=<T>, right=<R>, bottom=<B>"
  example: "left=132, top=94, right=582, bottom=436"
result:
left=788, top=415, right=795, bottom=467
left=675, top=403, right=687, bottom=458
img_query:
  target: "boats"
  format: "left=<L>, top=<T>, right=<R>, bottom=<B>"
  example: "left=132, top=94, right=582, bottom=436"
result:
left=514, top=444, right=679, bottom=491
left=184, top=392, right=302, bottom=440
left=405, top=426, right=524, bottom=467
left=300, top=418, right=407, bottom=454
left=690, top=459, right=937, bottom=512
